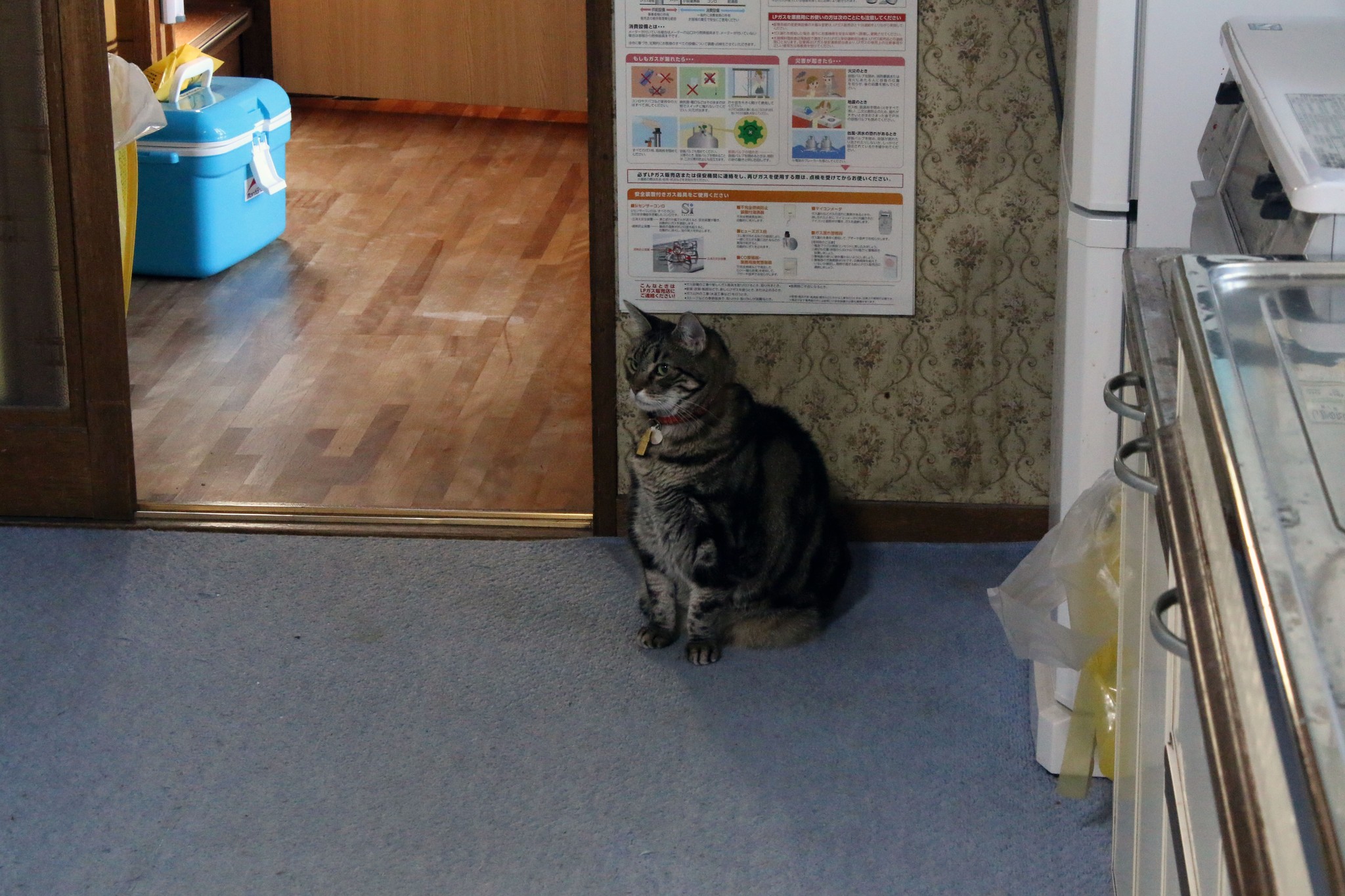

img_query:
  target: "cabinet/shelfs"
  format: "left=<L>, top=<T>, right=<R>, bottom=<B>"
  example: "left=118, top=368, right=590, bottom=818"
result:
left=118, top=1, right=253, bottom=82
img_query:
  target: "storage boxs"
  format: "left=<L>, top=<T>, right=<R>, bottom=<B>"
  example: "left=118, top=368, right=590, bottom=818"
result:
left=132, top=55, right=291, bottom=281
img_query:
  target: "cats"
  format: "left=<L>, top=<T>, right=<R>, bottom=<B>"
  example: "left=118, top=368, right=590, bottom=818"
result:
left=622, top=297, right=848, bottom=667
